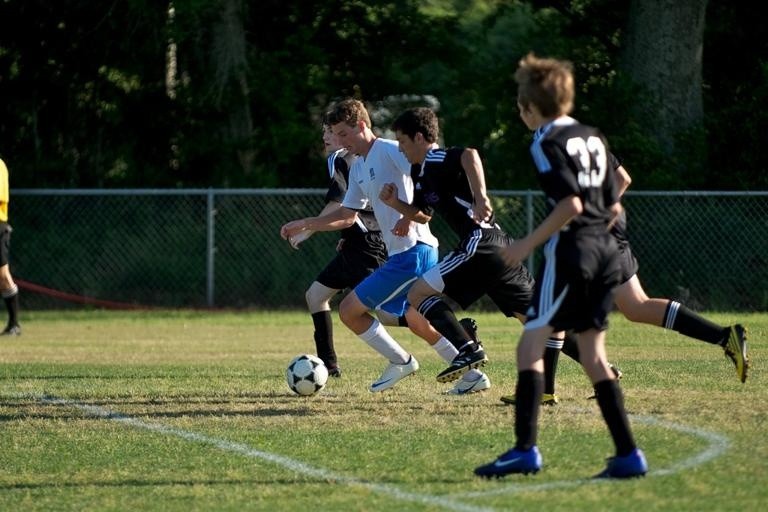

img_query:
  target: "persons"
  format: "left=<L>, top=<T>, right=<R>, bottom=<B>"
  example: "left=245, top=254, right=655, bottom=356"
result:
left=281, top=101, right=490, bottom=396
left=379, top=108, right=623, bottom=400
left=500, top=92, right=748, bottom=406
left=288, top=108, right=483, bottom=377
left=0, top=158, right=22, bottom=336
left=474, top=52, right=648, bottom=479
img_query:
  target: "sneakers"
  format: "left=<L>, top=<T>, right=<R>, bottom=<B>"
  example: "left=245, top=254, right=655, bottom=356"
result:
left=723, top=324, right=752, bottom=383
left=369, top=318, right=623, bottom=406
left=474, top=445, right=542, bottom=477
left=0, top=326, right=21, bottom=337
left=591, top=449, right=649, bottom=479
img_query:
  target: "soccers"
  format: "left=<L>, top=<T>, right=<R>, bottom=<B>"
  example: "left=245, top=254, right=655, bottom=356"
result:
left=287, top=355, right=329, bottom=396
